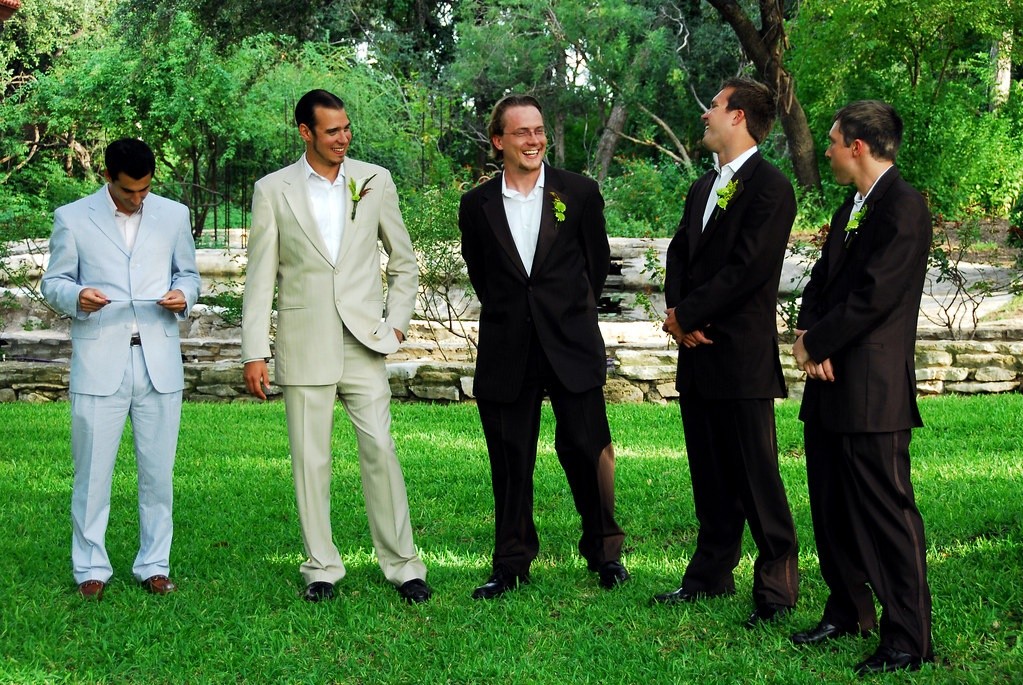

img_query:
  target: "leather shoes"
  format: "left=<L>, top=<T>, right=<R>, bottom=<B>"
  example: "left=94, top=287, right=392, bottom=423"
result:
left=79, top=580, right=105, bottom=602
left=304, top=581, right=334, bottom=602
left=471, top=576, right=528, bottom=600
left=791, top=620, right=878, bottom=648
left=597, top=560, right=630, bottom=590
left=142, top=574, right=176, bottom=594
left=744, top=610, right=771, bottom=630
left=653, top=586, right=698, bottom=605
left=854, top=647, right=934, bottom=679
left=399, top=578, right=431, bottom=605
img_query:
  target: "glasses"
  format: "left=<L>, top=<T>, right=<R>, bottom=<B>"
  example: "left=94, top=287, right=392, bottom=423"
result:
left=500, top=127, right=548, bottom=137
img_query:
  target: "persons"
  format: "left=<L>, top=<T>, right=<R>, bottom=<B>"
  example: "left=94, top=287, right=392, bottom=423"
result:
left=457, top=92, right=631, bottom=600
left=791, top=100, right=934, bottom=678
left=239, top=90, right=433, bottom=605
left=647, top=74, right=799, bottom=626
left=42, top=138, right=202, bottom=600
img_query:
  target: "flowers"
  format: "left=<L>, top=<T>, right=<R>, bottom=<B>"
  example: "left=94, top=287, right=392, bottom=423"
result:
left=348, top=173, right=376, bottom=220
left=551, top=191, right=566, bottom=229
left=844, top=204, right=869, bottom=232
left=715, top=179, right=739, bottom=209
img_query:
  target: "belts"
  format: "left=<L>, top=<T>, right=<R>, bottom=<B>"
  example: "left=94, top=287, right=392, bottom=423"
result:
left=129, top=336, right=141, bottom=346
